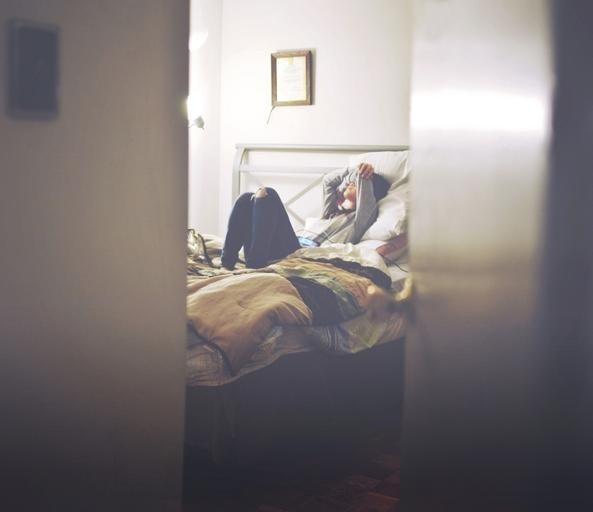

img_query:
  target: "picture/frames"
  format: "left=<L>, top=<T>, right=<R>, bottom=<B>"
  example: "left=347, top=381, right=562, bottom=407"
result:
left=268, top=49, right=314, bottom=107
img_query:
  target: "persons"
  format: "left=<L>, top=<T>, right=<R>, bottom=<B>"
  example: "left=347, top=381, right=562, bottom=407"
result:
left=220, top=162, right=389, bottom=272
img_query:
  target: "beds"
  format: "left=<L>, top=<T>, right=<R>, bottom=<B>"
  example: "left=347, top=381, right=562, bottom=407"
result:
left=185, top=140, right=411, bottom=386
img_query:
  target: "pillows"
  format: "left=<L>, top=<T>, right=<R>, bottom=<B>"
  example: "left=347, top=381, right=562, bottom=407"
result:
left=302, top=149, right=410, bottom=266
